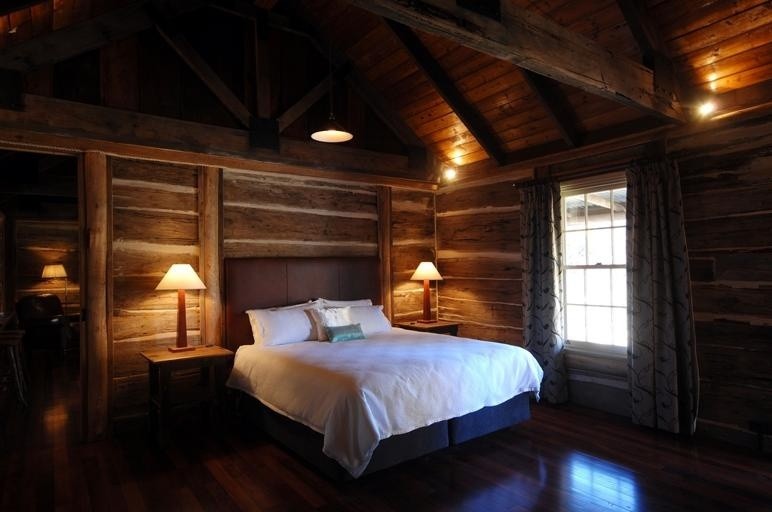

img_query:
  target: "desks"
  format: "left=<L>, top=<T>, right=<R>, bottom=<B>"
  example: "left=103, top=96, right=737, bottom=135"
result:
left=140, top=345, right=235, bottom=451
left=393, top=320, right=464, bottom=336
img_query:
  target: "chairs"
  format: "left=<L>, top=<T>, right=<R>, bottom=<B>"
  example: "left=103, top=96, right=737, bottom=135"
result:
left=15, top=293, right=72, bottom=361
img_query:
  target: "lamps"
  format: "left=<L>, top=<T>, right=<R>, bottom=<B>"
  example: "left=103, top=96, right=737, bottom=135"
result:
left=409, top=261, right=445, bottom=325
left=41, top=263, right=80, bottom=310
left=309, top=38, right=355, bottom=144
left=154, top=263, right=208, bottom=354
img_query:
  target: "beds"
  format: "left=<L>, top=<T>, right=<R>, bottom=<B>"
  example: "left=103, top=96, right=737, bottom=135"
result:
left=221, top=254, right=546, bottom=484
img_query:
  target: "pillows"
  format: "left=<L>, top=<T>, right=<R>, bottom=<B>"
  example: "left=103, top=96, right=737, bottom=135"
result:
left=244, top=297, right=393, bottom=346
